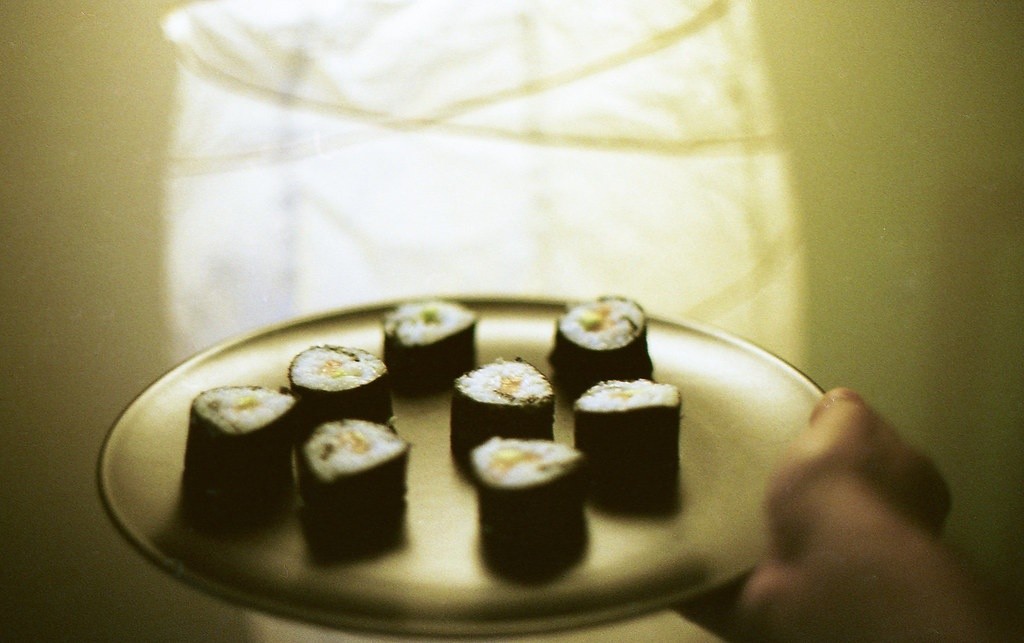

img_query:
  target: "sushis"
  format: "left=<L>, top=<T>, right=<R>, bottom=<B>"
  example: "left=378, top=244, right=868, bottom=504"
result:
left=462, top=428, right=586, bottom=502
left=375, top=297, right=481, bottom=401
left=285, top=343, right=392, bottom=432
left=181, top=383, right=299, bottom=487
left=565, top=378, right=685, bottom=463
left=442, top=355, right=558, bottom=445
left=548, top=291, right=658, bottom=396
left=298, top=417, right=414, bottom=516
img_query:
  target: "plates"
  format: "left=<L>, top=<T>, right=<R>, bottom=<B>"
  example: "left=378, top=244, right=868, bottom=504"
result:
left=99, top=299, right=827, bottom=637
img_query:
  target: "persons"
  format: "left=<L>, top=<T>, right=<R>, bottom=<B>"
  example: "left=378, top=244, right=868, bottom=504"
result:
left=680, top=385, right=1021, bottom=641
